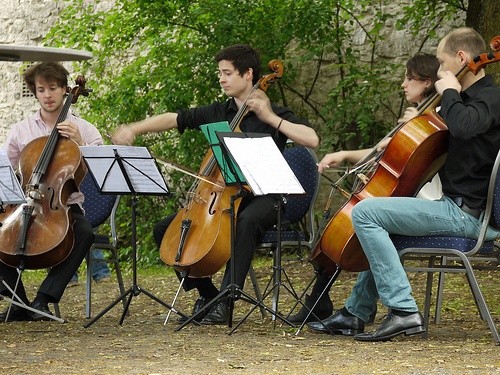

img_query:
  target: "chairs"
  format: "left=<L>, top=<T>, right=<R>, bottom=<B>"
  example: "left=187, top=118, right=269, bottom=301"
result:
left=50, top=159, right=130, bottom=320
left=230, top=138, right=321, bottom=319
left=384, top=148, right=499, bottom=345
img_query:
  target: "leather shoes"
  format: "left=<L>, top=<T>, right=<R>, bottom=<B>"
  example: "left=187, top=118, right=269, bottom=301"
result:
left=0, top=300, right=30, bottom=321
left=175, top=291, right=219, bottom=322
left=352, top=312, right=426, bottom=340
left=200, top=293, right=235, bottom=325
left=305, top=310, right=365, bottom=335
left=26, top=301, right=53, bottom=322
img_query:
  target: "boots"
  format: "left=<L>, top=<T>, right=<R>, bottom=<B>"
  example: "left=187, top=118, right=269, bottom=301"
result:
left=282, top=292, right=334, bottom=325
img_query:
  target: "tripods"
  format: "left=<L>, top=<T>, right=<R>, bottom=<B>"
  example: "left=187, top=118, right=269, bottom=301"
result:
left=175, top=122, right=298, bottom=335
left=215, top=131, right=334, bottom=335
left=78, top=144, right=200, bottom=330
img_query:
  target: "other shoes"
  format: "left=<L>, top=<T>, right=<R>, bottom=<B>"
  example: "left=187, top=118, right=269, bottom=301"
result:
left=65, top=277, right=113, bottom=288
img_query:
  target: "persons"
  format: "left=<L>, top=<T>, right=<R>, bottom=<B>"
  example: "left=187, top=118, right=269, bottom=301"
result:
left=285, top=51, right=445, bottom=325
left=307, top=26, right=500, bottom=342
left=111, top=46, right=319, bottom=325
left=0, top=62, right=104, bottom=322
left=67, top=244, right=113, bottom=287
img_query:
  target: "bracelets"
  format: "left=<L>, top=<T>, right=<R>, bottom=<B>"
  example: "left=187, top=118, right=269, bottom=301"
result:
left=276, top=119, right=284, bottom=131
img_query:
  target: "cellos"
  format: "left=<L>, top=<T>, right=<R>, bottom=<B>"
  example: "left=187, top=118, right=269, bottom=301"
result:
left=158, top=59, right=284, bottom=326
left=292, top=36, right=500, bottom=335
left=0, top=76, right=94, bottom=323
